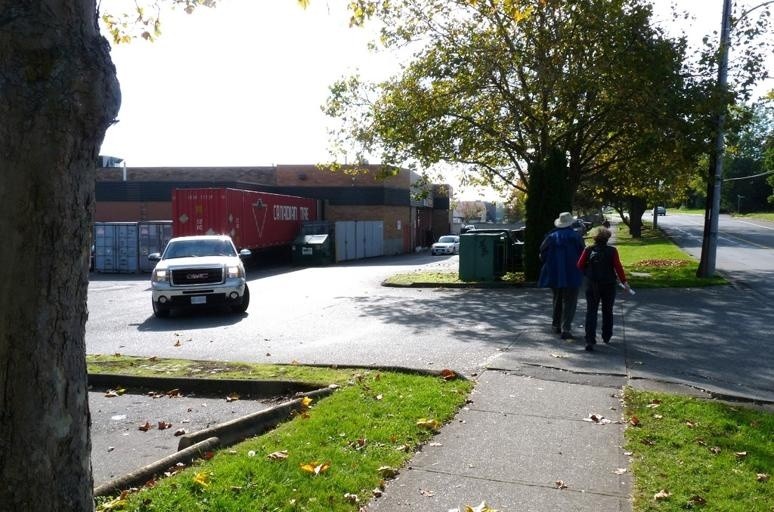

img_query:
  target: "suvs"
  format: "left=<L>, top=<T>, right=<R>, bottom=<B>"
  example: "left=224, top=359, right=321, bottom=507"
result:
left=145, top=233, right=252, bottom=319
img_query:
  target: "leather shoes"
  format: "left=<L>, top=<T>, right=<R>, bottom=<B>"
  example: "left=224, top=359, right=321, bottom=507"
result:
left=560, top=332, right=577, bottom=340
left=585, top=343, right=593, bottom=350
left=603, top=338, right=609, bottom=343
left=552, top=326, right=561, bottom=334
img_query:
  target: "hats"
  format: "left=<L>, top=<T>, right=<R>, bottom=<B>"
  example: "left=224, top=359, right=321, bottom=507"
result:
left=554, top=212, right=578, bottom=228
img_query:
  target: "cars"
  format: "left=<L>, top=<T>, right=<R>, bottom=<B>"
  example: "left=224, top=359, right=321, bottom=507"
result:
left=429, top=235, right=460, bottom=256
left=572, top=216, right=593, bottom=236
left=652, top=207, right=666, bottom=216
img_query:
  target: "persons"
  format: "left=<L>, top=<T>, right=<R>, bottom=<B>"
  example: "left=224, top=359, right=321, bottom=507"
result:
left=574, top=225, right=630, bottom=353
left=601, top=218, right=610, bottom=230
left=536, top=212, right=588, bottom=340
